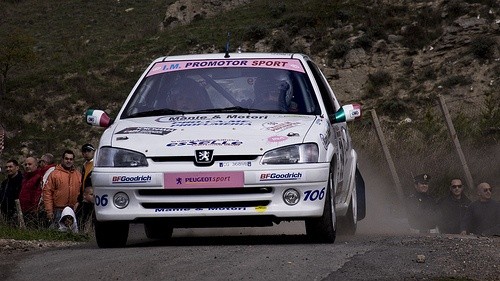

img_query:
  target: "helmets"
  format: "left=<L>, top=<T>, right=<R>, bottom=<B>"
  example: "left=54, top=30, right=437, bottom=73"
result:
left=255, top=71, right=293, bottom=106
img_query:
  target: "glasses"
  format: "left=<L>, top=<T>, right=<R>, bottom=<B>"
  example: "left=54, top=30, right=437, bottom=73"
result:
left=39, top=160, right=45, bottom=163
left=83, top=149, right=91, bottom=153
left=64, top=157, right=74, bottom=161
left=479, top=187, right=492, bottom=192
left=452, top=185, right=462, bottom=189
left=63, top=216, right=73, bottom=221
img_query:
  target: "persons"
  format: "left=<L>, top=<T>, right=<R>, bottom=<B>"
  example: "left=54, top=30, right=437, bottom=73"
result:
left=241, top=72, right=298, bottom=113
left=43, top=150, right=82, bottom=228
left=472, top=183, right=500, bottom=234
left=74, top=143, right=96, bottom=212
left=407, top=174, right=439, bottom=229
left=439, top=177, right=473, bottom=233
left=77, top=187, right=94, bottom=230
left=38, top=153, right=55, bottom=227
left=19, top=156, right=42, bottom=228
left=173, top=85, right=203, bottom=112
left=59, top=206, right=78, bottom=232
left=0, top=160, right=23, bottom=221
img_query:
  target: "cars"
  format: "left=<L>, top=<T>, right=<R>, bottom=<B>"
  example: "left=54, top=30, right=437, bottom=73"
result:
left=84, top=53, right=366, bottom=248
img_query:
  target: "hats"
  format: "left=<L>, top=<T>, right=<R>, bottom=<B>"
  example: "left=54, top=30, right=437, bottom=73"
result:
left=81, top=144, right=95, bottom=151
left=414, top=174, right=431, bottom=182
